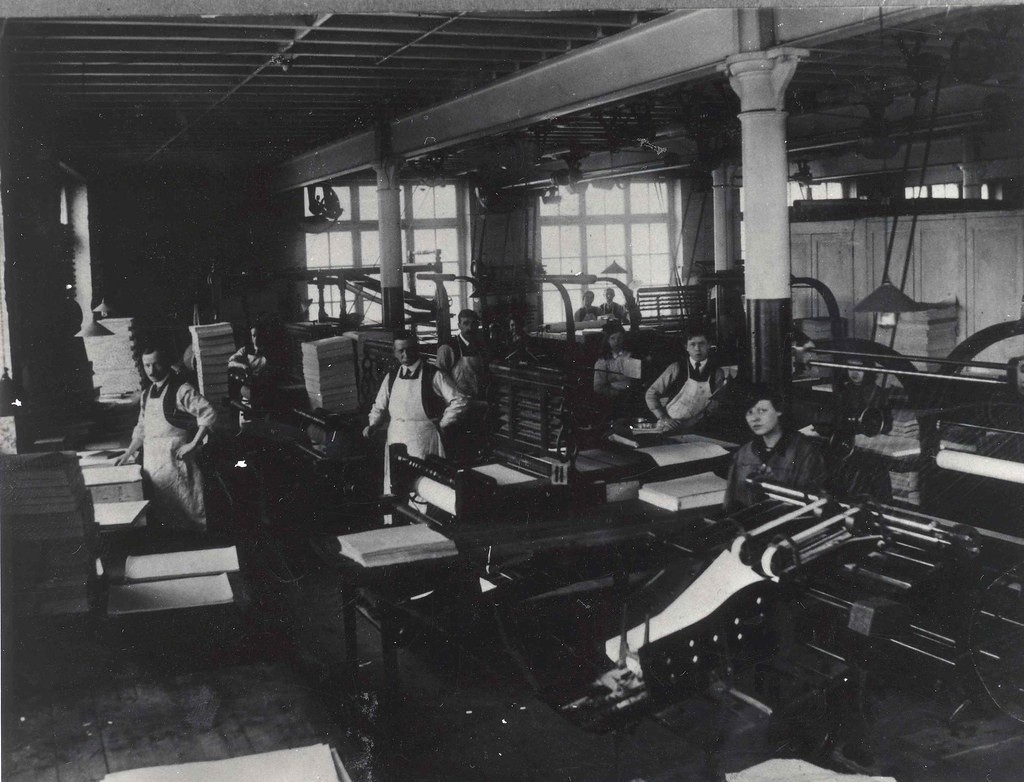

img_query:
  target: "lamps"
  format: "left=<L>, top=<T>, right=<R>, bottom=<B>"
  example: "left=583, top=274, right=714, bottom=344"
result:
left=851, top=159, right=928, bottom=327
left=600, top=259, right=628, bottom=274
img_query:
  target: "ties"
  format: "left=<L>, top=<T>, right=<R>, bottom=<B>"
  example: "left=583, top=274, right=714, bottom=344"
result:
left=256, top=350, right=262, bottom=357
left=405, top=368, right=412, bottom=378
left=608, top=304, right=611, bottom=310
left=468, top=344, right=477, bottom=356
left=694, top=362, right=701, bottom=376
left=150, top=384, right=157, bottom=398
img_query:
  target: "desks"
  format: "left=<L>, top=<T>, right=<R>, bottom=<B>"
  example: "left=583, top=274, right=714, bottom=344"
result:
left=336, top=499, right=732, bottom=711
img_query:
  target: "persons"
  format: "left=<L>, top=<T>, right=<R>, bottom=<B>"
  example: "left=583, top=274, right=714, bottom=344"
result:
left=574, top=289, right=628, bottom=328
left=507, top=316, right=536, bottom=362
left=726, top=388, right=831, bottom=499
left=837, top=351, right=894, bottom=434
left=644, top=331, right=727, bottom=429
left=228, top=325, right=270, bottom=438
left=363, top=333, right=470, bottom=528
left=594, top=325, right=643, bottom=396
left=437, top=308, right=488, bottom=457
left=116, top=343, right=217, bottom=548
